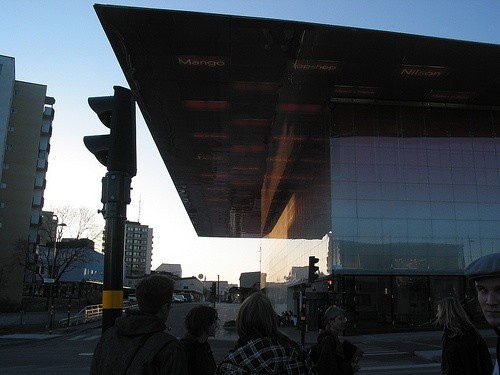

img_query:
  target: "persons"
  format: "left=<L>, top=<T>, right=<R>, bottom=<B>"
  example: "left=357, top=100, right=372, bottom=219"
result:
left=441, top=302, right=494, bottom=375
left=179, top=305, right=219, bottom=375
left=311, top=304, right=360, bottom=375
left=89, top=275, right=188, bottom=375
left=282, top=310, right=294, bottom=329
left=465, top=252, right=500, bottom=375
left=215, top=292, right=318, bottom=375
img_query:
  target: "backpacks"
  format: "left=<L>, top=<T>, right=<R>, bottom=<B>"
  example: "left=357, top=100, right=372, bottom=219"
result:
left=309, top=336, right=335, bottom=364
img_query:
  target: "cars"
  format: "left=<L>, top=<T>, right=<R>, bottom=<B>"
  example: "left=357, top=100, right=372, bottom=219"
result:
left=172, top=292, right=194, bottom=303
left=128, top=293, right=136, bottom=302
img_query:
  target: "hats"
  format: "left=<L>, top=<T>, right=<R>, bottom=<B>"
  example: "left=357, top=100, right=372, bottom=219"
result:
left=464, top=253, right=500, bottom=280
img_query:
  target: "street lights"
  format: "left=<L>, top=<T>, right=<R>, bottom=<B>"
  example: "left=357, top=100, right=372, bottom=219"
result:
left=47, top=223, right=66, bottom=328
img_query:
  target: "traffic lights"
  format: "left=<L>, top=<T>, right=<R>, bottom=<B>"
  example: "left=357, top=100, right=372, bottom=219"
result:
left=328, top=278, right=334, bottom=287
left=210, top=283, right=216, bottom=294
left=309, top=256, right=319, bottom=282
left=82, top=85, right=137, bottom=177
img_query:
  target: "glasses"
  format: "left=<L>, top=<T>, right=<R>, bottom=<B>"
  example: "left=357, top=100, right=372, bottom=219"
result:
left=335, top=317, right=348, bottom=322
